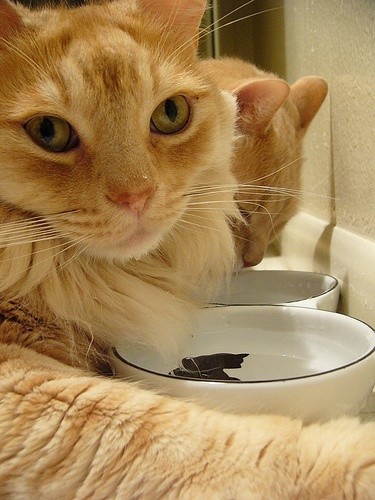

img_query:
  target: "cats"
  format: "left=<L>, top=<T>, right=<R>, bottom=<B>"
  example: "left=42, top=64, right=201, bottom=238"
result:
left=1, top=0, right=374, bottom=500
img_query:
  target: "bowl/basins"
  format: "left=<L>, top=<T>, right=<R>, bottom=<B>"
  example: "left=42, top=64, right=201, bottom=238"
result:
left=204, top=269, right=340, bottom=313
left=105, top=304, right=375, bottom=425
